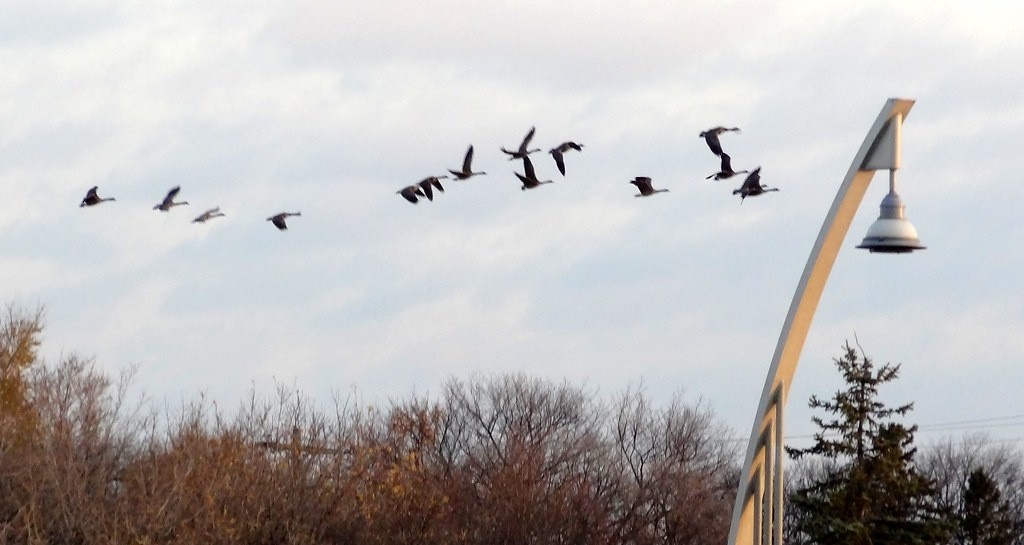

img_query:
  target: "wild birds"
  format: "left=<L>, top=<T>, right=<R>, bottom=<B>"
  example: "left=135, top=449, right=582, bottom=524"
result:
left=151, top=185, right=190, bottom=212
left=78, top=185, right=116, bottom=208
left=699, top=125, right=781, bottom=205
left=191, top=205, right=225, bottom=225
left=630, top=177, right=670, bottom=199
left=266, top=210, right=301, bottom=232
left=394, top=144, right=489, bottom=204
left=498, top=124, right=586, bottom=191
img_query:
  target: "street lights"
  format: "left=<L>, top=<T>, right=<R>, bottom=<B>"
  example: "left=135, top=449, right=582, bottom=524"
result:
left=725, top=95, right=930, bottom=542
